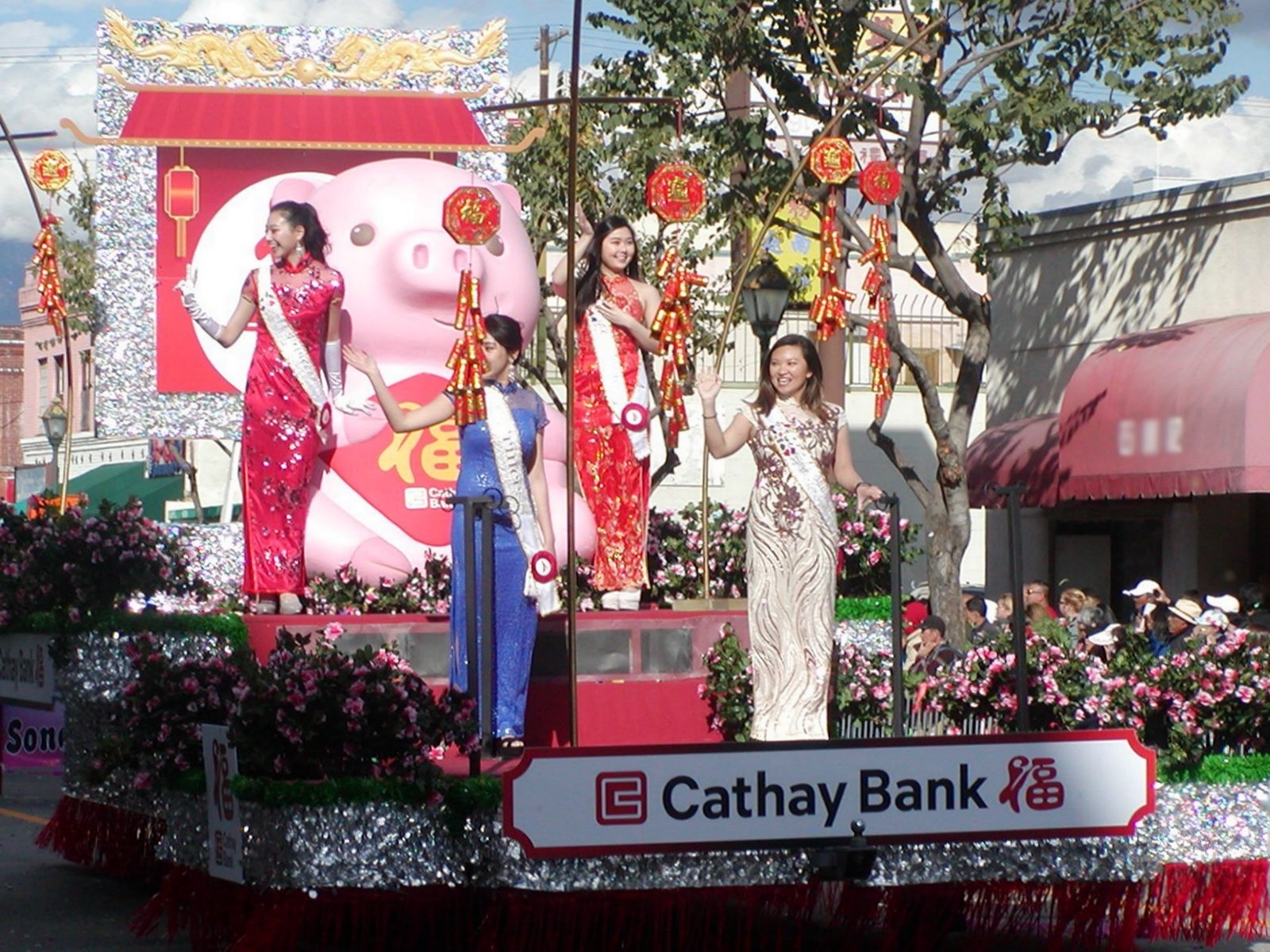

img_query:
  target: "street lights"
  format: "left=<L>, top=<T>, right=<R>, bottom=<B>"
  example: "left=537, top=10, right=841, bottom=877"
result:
left=39, top=394, right=69, bottom=496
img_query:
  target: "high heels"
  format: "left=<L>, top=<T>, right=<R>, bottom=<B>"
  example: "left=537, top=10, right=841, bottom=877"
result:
left=617, top=590, right=641, bottom=610
left=258, top=595, right=276, bottom=613
left=601, top=590, right=618, bottom=611
left=495, top=736, right=525, bottom=757
left=279, top=598, right=303, bottom=615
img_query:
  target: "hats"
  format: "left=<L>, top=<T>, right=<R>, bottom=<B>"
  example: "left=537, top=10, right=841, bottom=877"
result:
left=1167, top=598, right=1203, bottom=625
left=1206, top=594, right=1240, bottom=614
left=1197, top=608, right=1229, bottom=630
left=1088, top=623, right=1124, bottom=645
left=913, top=614, right=946, bottom=633
left=1130, top=580, right=1160, bottom=596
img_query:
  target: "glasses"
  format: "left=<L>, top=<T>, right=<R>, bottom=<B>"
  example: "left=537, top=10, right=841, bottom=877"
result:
left=1058, top=599, right=1068, bottom=604
left=1027, top=589, right=1044, bottom=595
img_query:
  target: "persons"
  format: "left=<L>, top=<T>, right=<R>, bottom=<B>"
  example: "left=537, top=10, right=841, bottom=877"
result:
left=1025, top=580, right=1058, bottom=619
left=901, top=602, right=928, bottom=669
left=1024, top=603, right=1046, bottom=638
left=1059, top=576, right=1118, bottom=663
left=1130, top=580, right=1270, bottom=667
left=964, top=596, right=998, bottom=646
left=172, top=200, right=377, bottom=615
left=698, top=333, right=883, bottom=741
left=552, top=202, right=662, bottom=611
left=909, top=616, right=962, bottom=677
left=343, top=313, right=555, bottom=756
left=993, top=592, right=1013, bottom=634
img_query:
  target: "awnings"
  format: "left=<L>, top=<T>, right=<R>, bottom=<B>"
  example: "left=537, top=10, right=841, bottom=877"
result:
left=13, top=461, right=184, bottom=525
left=964, top=415, right=1109, bottom=510
left=1058, top=311, right=1270, bottom=500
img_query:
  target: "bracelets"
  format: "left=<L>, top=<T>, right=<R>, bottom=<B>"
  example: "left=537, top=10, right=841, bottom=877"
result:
left=854, top=482, right=871, bottom=494
left=703, top=415, right=716, bottom=419
